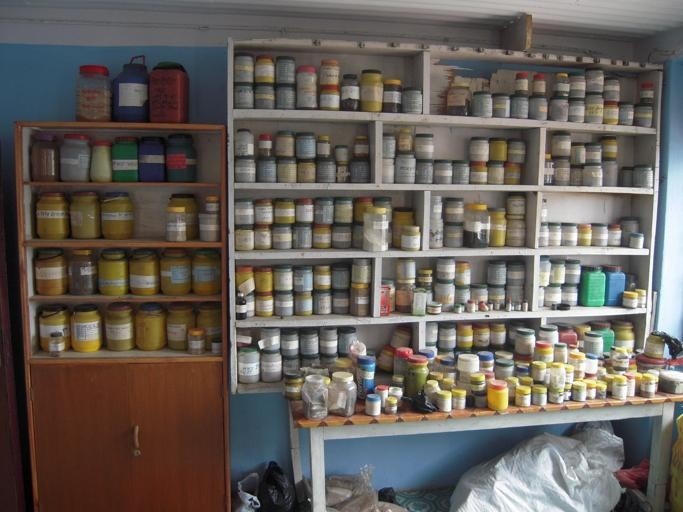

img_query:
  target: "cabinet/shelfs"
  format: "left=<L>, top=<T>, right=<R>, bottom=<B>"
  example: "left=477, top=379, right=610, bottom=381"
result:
left=12, top=117, right=227, bottom=360
left=222, top=35, right=666, bottom=396
left=20, top=359, right=231, bottom=512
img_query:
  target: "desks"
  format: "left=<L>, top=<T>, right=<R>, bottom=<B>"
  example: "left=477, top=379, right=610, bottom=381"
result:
left=282, top=386, right=682, bottom=512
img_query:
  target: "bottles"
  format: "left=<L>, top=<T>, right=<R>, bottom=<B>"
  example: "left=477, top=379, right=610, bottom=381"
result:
left=544, top=132, right=654, bottom=187
left=212, top=336, right=221, bottom=354
left=446, top=67, right=654, bottom=128
left=90, top=141, right=111, bottom=182
left=541, top=256, right=647, bottom=310
left=381, top=258, right=528, bottom=315
left=234, top=52, right=422, bottom=113
left=235, top=259, right=371, bottom=318
left=538, top=197, right=644, bottom=249
left=234, top=125, right=370, bottom=183
left=237, top=320, right=667, bottom=421
left=234, top=196, right=420, bottom=252
left=189, top=328, right=205, bottom=354
left=166, top=206, right=186, bottom=242
left=383, top=127, right=527, bottom=185
left=49, top=331, right=65, bottom=357
left=430, top=195, right=527, bottom=248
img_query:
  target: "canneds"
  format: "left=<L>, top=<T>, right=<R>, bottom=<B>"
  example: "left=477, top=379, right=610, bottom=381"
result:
left=383, top=79, right=402, bottom=112
